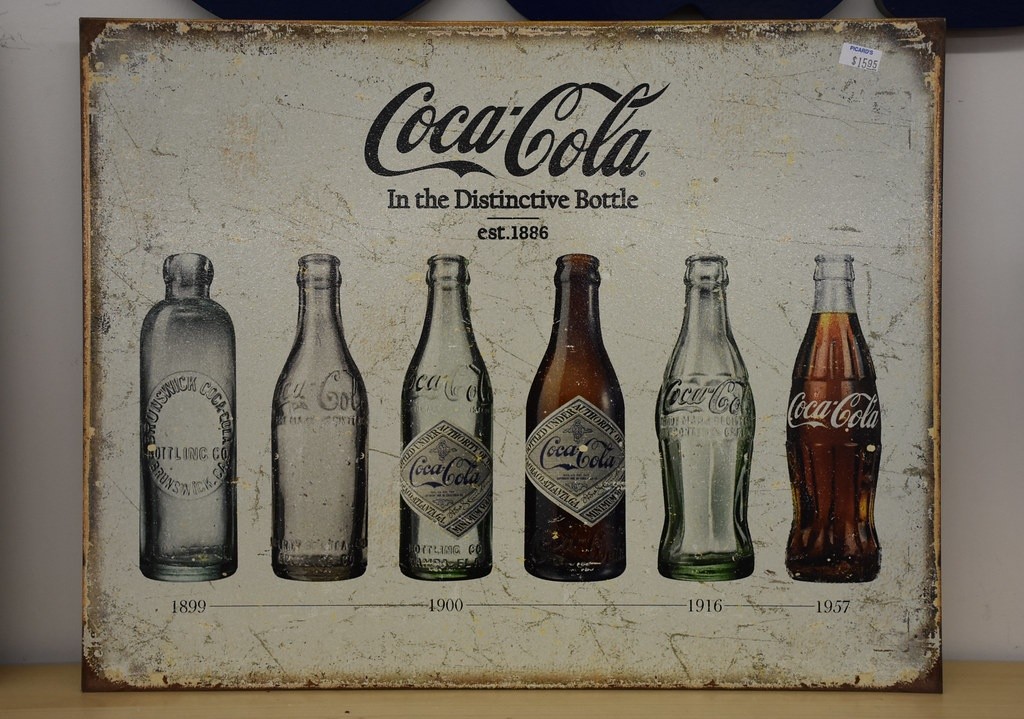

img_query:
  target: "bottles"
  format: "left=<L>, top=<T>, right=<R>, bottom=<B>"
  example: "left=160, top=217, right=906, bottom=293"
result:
left=654, top=254, right=758, bottom=582
left=399, top=254, right=495, bottom=582
left=138, top=253, right=237, bottom=583
left=525, top=253, right=629, bottom=583
left=785, top=252, right=882, bottom=585
left=271, top=252, right=371, bottom=581
